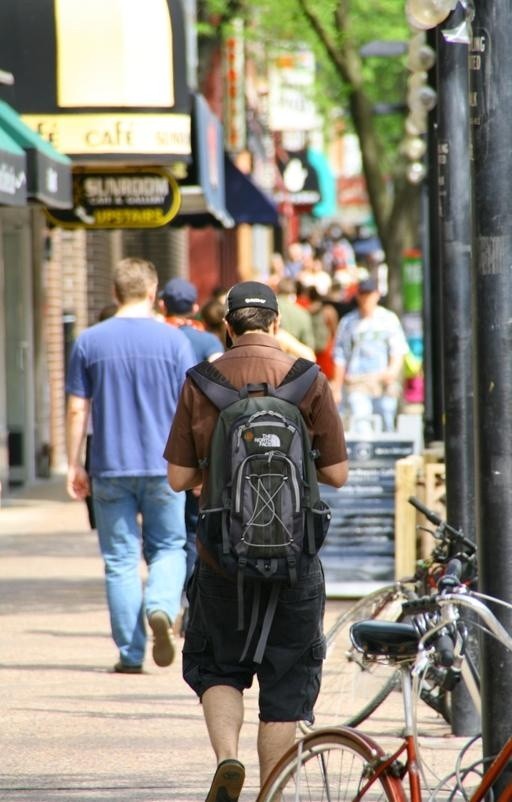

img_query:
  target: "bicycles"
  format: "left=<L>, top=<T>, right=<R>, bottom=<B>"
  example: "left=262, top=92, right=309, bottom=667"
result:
left=255, top=591, right=512, bottom=802
left=324, top=500, right=480, bottom=737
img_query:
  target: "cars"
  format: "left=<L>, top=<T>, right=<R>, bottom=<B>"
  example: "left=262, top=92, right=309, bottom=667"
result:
left=354, top=220, right=389, bottom=269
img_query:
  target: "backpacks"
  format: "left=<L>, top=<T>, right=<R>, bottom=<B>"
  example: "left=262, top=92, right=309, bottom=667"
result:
left=186, top=358, right=331, bottom=589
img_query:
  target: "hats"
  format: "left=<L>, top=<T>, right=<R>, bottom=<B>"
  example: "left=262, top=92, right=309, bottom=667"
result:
left=158, top=278, right=195, bottom=303
left=226, top=281, right=278, bottom=347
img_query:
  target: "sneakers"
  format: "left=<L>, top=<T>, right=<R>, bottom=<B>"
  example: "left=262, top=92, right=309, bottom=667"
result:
left=204, top=759, right=245, bottom=802
left=149, top=611, right=174, bottom=666
left=115, top=662, right=140, bottom=673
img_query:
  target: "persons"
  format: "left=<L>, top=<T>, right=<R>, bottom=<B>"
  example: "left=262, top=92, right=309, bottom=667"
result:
left=80, top=303, right=118, bottom=532
left=162, top=278, right=350, bottom=802
left=156, top=275, right=226, bottom=639
left=66, top=257, right=198, bottom=675
